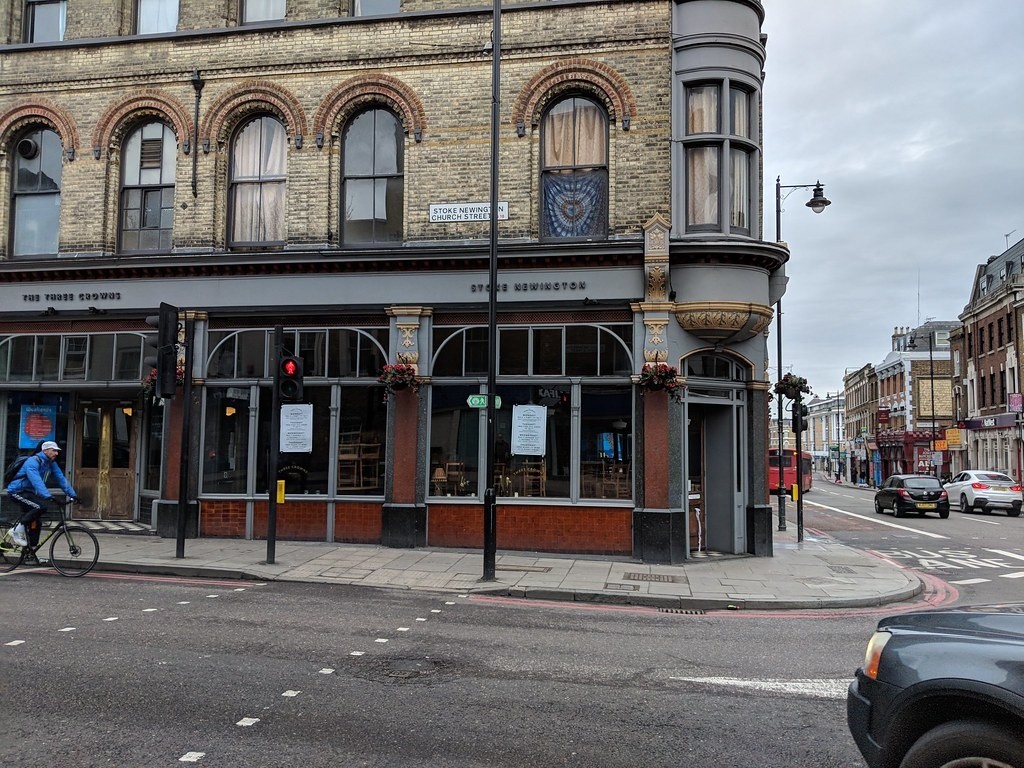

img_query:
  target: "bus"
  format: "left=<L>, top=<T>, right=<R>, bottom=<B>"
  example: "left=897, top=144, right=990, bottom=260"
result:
left=769, top=448, right=814, bottom=494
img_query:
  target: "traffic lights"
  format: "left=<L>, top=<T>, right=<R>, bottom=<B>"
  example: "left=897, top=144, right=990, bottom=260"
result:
left=792, top=403, right=808, bottom=433
left=143, top=302, right=179, bottom=398
left=279, top=357, right=304, bottom=404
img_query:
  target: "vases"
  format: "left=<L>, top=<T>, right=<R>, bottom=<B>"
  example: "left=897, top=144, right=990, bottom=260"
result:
left=391, top=384, right=409, bottom=391
left=783, top=389, right=805, bottom=399
left=650, top=383, right=665, bottom=391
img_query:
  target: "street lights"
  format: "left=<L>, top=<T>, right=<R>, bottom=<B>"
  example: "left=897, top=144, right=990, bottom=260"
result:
left=906, top=331, right=936, bottom=452
left=827, top=393, right=841, bottom=480
left=775, top=176, right=831, bottom=544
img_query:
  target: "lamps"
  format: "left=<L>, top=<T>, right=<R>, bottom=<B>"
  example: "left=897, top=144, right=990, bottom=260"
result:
left=431, top=468, right=447, bottom=496
left=47, top=307, right=56, bottom=315
left=88, top=306, right=100, bottom=315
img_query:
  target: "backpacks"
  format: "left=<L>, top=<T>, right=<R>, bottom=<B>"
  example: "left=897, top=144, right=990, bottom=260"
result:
left=5, top=454, right=42, bottom=488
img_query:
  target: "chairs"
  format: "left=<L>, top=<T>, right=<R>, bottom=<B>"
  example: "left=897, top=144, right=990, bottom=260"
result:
left=520, top=458, right=543, bottom=496
left=445, top=463, right=465, bottom=496
left=338, top=431, right=381, bottom=486
left=578, top=456, right=631, bottom=500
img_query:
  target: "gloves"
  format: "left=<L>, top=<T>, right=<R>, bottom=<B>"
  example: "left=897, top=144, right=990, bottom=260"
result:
left=73, top=497, right=83, bottom=504
left=47, top=496, right=56, bottom=501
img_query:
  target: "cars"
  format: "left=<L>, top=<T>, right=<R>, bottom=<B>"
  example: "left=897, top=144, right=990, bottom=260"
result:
left=874, top=475, right=950, bottom=519
left=943, top=469, right=1023, bottom=517
left=845, top=603, right=1024, bottom=768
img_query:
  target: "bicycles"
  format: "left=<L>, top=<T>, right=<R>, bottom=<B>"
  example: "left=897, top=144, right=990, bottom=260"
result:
left=0, top=498, right=100, bottom=577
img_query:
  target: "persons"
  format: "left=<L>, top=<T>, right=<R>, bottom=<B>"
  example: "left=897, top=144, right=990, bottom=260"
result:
left=7, top=440, right=84, bottom=565
left=859, top=469, right=867, bottom=484
left=495, top=433, right=510, bottom=472
left=869, top=478, right=874, bottom=487
left=852, top=466, right=858, bottom=485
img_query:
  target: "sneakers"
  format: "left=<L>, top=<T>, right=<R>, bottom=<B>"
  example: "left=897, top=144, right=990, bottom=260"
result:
left=8, top=527, right=27, bottom=546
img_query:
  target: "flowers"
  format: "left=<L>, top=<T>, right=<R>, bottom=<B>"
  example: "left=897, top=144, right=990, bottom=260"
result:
left=380, top=363, right=414, bottom=382
left=642, top=362, right=687, bottom=404
left=774, top=371, right=808, bottom=389
left=139, top=368, right=183, bottom=397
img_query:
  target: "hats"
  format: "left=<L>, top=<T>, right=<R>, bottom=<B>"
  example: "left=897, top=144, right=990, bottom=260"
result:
left=42, top=441, right=61, bottom=450
